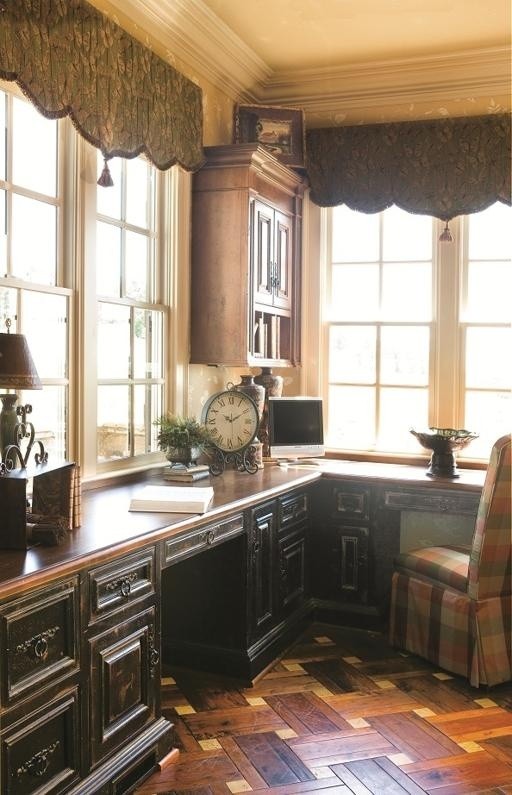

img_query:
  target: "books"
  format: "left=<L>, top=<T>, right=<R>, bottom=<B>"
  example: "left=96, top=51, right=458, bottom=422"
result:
left=30, top=465, right=82, bottom=531
left=128, top=485, right=215, bottom=514
left=254, top=315, right=280, bottom=360
left=162, top=464, right=210, bottom=482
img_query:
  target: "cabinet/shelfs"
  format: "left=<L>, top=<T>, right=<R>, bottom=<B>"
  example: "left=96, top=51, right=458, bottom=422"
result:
left=1, top=544, right=175, bottom=795
left=242, top=486, right=316, bottom=686
left=314, top=477, right=376, bottom=628
left=189, top=143, right=302, bottom=369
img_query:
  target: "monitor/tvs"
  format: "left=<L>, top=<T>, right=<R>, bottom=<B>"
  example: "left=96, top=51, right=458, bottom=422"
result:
left=269, top=397, right=325, bottom=459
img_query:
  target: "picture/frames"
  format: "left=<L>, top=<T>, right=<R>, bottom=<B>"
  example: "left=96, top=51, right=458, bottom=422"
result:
left=236, top=103, right=306, bottom=170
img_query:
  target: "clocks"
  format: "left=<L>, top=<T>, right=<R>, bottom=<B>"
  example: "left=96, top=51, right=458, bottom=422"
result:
left=200, top=381, right=259, bottom=477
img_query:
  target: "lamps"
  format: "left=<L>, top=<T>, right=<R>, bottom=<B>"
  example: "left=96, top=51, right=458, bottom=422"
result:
left=0, top=318, right=43, bottom=472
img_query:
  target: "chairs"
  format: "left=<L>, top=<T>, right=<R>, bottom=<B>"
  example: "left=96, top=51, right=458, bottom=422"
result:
left=387, top=435, right=512, bottom=692
